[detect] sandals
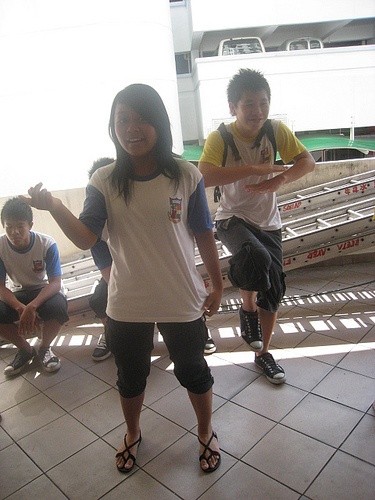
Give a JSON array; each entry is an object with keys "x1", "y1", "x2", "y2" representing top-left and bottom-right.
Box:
[
  {"x1": 114, "y1": 429, "x2": 143, "y2": 474},
  {"x1": 197, "y1": 430, "x2": 221, "y2": 472}
]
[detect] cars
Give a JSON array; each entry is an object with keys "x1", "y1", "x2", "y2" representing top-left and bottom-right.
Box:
[
  {"x1": 218, "y1": 37, "x2": 266, "y2": 56},
  {"x1": 285, "y1": 37, "x2": 323, "y2": 51}
]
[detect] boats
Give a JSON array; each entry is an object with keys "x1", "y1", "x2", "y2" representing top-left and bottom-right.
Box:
[{"x1": 275, "y1": 147, "x2": 375, "y2": 165}]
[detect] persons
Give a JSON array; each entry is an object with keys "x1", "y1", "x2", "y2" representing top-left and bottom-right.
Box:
[
  {"x1": 80, "y1": 158, "x2": 218, "y2": 362},
  {"x1": 19, "y1": 84, "x2": 223, "y2": 476},
  {"x1": 0, "y1": 197, "x2": 70, "y2": 377},
  {"x1": 198, "y1": 68, "x2": 318, "y2": 384}
]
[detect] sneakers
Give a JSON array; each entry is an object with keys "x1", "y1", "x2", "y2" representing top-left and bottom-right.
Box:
[
  {"x1": 254, "y1": 351, "x2": 286, "y2": 384},
  {"x1": 92, "y1": 333, "x2": 111, "y2": 361},
  {"x1": 3, "y1": 346, "x2": 35, "y2": 376},
  {"x1": 37, "y1": 347, "x2": 61, "y2": 372},
  {"x1": 205, "y1": 329, "x2": 217, "y2": 354},
  {"x1": 239, "y1": 305, "x2": 264, "y2": 353}
]
